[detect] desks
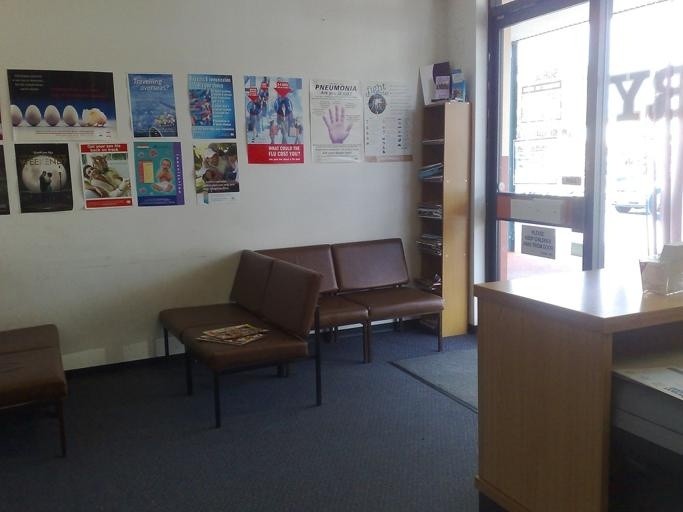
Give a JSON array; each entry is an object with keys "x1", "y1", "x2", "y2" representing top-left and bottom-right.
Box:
[{"x1": 467, "y1": 263, "x2": 682, "y2": 512}]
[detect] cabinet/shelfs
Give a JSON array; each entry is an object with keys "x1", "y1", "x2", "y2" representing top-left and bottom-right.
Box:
[{"x1": 413, "y1": 100, "x2": 470, "y2": 338}]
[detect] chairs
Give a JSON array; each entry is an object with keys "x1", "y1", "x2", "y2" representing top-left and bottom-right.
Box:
[
  {"x1": 254, "y1": 236, "x2": 369, "y2": 364},
  {"x1": 180, "y1": 258, "x2": 325, "y2": 427},
  {"x1": 331, "y1": 238, "x2": 444, "y2": 362},
  {"x1": 158, "y1": 248, "x2": 275, "y2": 398}
]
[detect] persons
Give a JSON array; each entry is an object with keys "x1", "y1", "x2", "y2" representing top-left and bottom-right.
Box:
[
  {"x1": 247, "y1": 92, "x2": 301, "y2": 144},
  {"x1": 84, "y1": 156, "x2": 131, "y2": 197},
  {"x1": 152, "y1": 160, "x2": 174, "y2": 191},
  {"x1": 40, "y1": 171, "x2": 52, "y2": 192},
  {"x1": 322, "y1": 105, "x2": 353, "y2": 144}
]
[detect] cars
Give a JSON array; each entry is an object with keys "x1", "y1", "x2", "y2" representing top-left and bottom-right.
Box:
[{"x1": 609, "y1": 173, "x2": 661, "y2": 218}]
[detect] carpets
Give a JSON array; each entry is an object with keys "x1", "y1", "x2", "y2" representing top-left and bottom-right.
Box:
[{"x1": 387, "y1": 346, "x2": 480, "y2": 415}]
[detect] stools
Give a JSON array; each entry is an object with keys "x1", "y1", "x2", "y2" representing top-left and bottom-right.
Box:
[{"x1": 0, "y1": 323, "x2": 69, "y2": 454}]
